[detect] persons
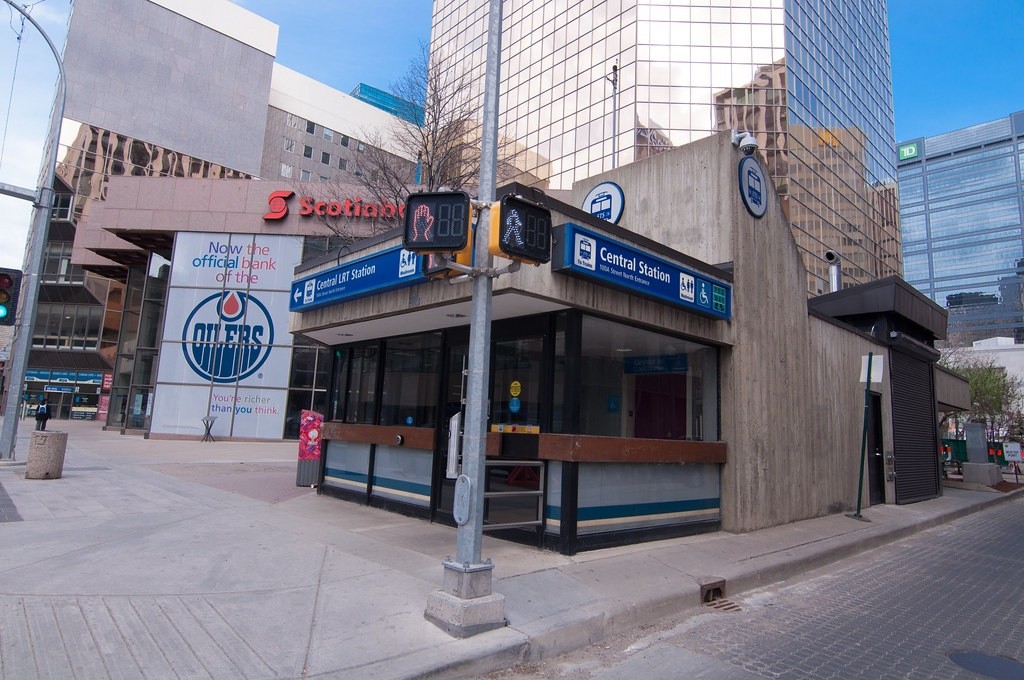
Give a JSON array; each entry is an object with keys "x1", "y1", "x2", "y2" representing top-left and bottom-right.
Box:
[{"x1": 35, "y1": 398, "x2": 51, "y2": 431}]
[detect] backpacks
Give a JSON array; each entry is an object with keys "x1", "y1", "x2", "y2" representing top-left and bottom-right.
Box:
[{"x1": 37, "y1": 404, "x2": 48, "y2": 414}]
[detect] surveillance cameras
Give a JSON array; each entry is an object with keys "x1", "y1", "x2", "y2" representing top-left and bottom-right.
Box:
[{"x1": 739, "y1": 136, "x2": 759, "y2": 156}]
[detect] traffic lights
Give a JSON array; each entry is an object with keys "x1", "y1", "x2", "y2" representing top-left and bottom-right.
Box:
[
  {"x1": 418, "y1": 247, "x2": 472, "y2": 277},
  {"x1": 0, "y1": 267, "x2": 23, "y2": 326},
  {"x1": 488, "y1": 194, "x2": 553, "y2": 266},
  {"x1": 402, "y1": 191, "x2": 473, "y2": 253}
]
